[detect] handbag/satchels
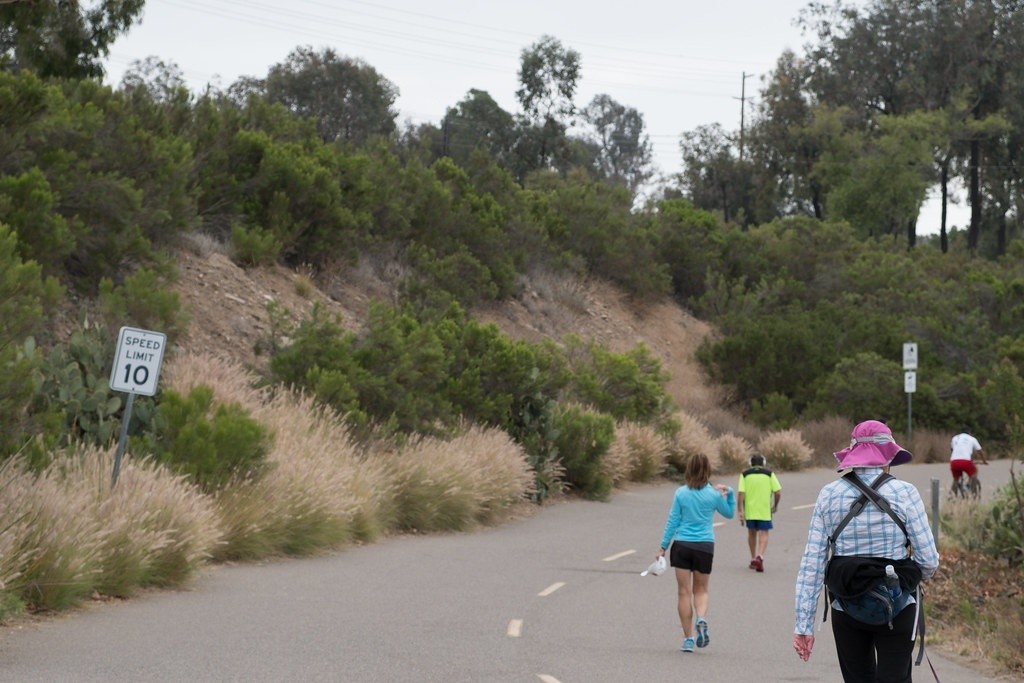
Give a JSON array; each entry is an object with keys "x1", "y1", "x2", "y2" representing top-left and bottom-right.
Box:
[{"x1": 833, "y1": 581, "x2": 910, "y2": 626}]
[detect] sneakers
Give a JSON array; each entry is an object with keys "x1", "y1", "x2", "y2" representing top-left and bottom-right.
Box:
[
  {"x1": 680, "y1": 638, "x2": 694, "y2": 652},
  {"x1": 755, "y1": 556, "x2": 764, "y2": 572},
  {"x1": 750, "y1": 559, "x2": 755, "y2": 569},
  {"x1": 696, "y1": 616, "x2": 710, "y2": 648}
]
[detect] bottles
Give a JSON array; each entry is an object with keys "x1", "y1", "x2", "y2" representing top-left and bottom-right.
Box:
[{"x1": 885, "y1": 564, "x2": 902, "y2": 602}]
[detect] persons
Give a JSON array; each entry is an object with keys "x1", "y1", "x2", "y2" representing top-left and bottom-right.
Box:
[
  {"x1": 949, "y1": 426, "x2": 989, "y2": 496},
  {"x1": 656, "y1": 452, "x2": 736, "y2": 652},
  {"x1": 738, "y1": 453, "x2": 781, "y2": 572},
  {"x1": 793, "y1": 420, "x2": 940, "y2": 683}
]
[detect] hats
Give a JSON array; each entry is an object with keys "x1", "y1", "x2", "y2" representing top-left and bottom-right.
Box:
[
  {"x1": 640, "y1": 556, "x2": 667, "y2": 576},
  {"x1": 833, "y1": 420, "x2": 913, "y2": 474}
]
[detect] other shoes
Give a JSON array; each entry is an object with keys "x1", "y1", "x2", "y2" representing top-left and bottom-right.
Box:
[{"x1": 963, "y1": 483, "x2": 970, "y2": 492}]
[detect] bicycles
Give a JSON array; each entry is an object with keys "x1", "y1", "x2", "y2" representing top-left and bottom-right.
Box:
[{"x1": 948, "y1": 462, "x2": 989, "y2": 501}]
